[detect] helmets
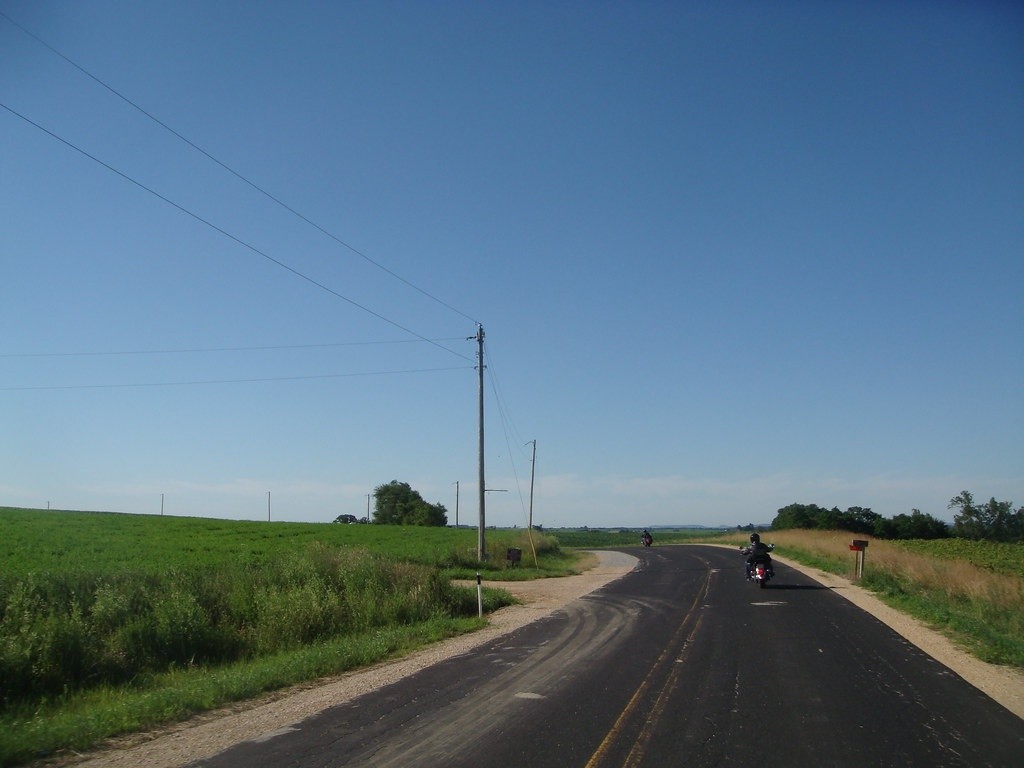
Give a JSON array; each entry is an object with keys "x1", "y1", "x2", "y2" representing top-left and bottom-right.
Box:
[
  {"x1": 643, "y1": 530, "x2": 646, "y2": 532},
  {"x1": 750, "y1": 533, "x2": 760, "y2": 543}
]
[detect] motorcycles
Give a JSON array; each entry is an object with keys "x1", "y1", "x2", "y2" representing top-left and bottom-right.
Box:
[
  {"x1": 641, "y1": 535, "x2": 652, "y2": 547},
  {"x1": 738, "y1": 544, "x2": 776, "y2": 588}
]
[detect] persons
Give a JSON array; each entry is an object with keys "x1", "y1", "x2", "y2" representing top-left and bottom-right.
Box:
[
  {"x1": 641, "y1": 529, "x2": 652, "y2": 543},
  {"x1": 742, "y1": 533, "x2": 773, "y2": 576}
]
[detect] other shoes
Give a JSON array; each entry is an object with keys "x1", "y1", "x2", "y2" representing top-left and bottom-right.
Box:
[{"x1": 744, "y1": 573, "x2": 749, "y2": 576}]
